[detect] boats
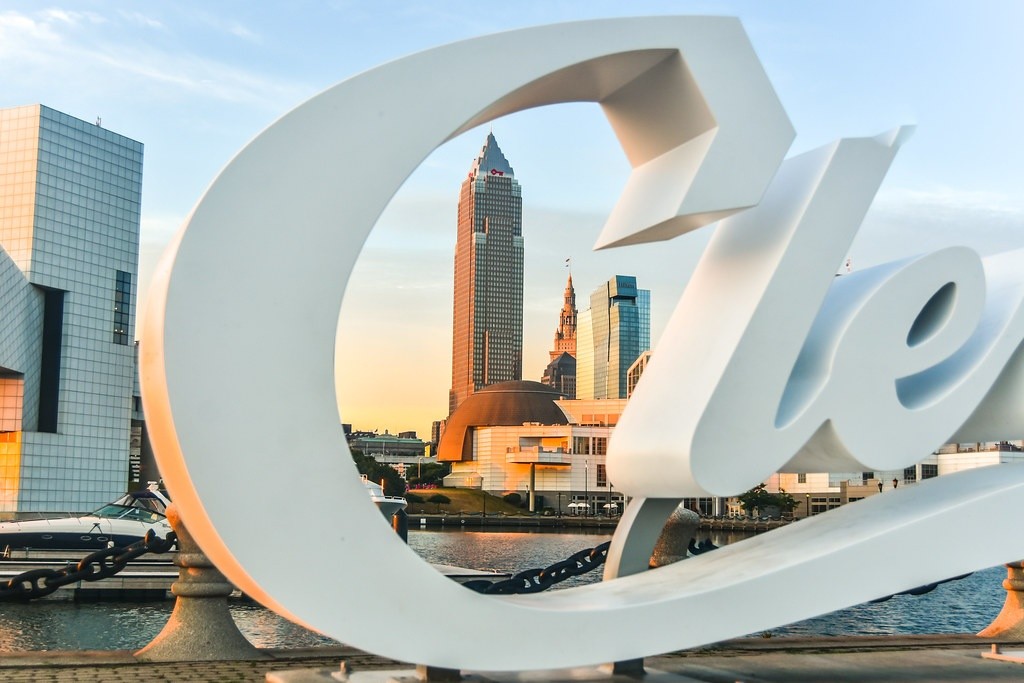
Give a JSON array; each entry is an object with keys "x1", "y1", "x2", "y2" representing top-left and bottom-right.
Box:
[
  {"x1": 427, "y1": 563, "x2": 512, "y2": 584},
  {"x1": 0, "y1": 475, "x2": 409, "y2": 593}
]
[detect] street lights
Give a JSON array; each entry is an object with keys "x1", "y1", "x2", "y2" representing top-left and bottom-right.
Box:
[
  {"x1": 805, "y1": 493, "x2": 811, "y2": 517},
  {"x1": 558, "y1": 492, "x2": 562, "y2": 517},
  {"x1": 609, "y1": 482, "x2": 614, "y2": 518},
  {"x1": 482, "y1": 489, "x2": 486, "y2": 516}
]
